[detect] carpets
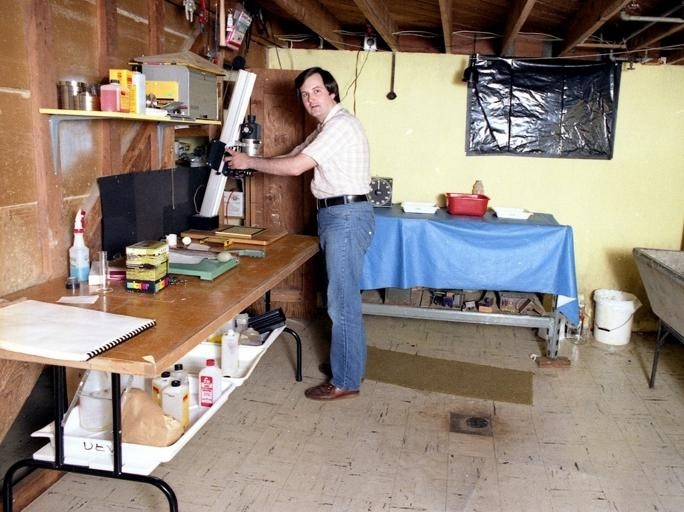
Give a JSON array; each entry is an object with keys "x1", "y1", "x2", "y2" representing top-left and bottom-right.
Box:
[{"x1": 366, "y1": 344, "x2": 534, "y2": 406}]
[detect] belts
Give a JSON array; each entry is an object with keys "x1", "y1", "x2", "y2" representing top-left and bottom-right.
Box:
[{"x1": 316, "y1": 194, "x2": 366, "y2": 210}]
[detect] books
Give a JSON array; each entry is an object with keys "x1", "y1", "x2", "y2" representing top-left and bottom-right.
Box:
[{"x1": 0, "y1": 300, "x2": 157, "y2": 362}]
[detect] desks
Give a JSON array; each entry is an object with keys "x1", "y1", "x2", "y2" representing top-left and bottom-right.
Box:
[
  {"x1": 0, "y1": 232, "x2": 320, "y2": 512},
  {"x1": 360, "y1": 202, "x2": 580, "y2": 367}
]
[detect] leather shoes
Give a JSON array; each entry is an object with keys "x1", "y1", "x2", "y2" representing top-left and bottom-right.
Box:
[
  {"x1": 305, "y1": 381, "x2": 360, "y2": 401},
  {"x1": 319, "y1": 362, "x2": 364, "y2": 382}
]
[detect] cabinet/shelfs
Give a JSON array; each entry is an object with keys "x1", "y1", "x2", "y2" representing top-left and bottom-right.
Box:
[{"x1": 243, "y1": 67, "x2": 324, "y2": 321}]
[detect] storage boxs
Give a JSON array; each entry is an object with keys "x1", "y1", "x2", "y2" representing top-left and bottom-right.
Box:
[{"x1": 142, "y1": 63, "x2": 218, "y2": 120}]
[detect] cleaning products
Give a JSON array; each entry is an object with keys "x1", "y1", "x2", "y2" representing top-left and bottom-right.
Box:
[
  {"x1": 154, "y1": 330, "x2": 240, "y2": 430},
  {"x1": 68, "y1": 209, "x2": 90, "y2": 282},
  {"x1": 132, "y1": 64, "x2": 146, "y2": 114}
]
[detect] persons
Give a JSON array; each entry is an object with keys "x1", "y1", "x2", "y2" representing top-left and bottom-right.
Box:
[{"x1": 225, "y1": 67, "x2": 376, "y2": 400}]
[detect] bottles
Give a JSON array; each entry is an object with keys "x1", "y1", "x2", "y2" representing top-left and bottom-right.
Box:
[
  {"x1": 221, "y1": 329, "x2": 240, "y2": 377},
  {"x1": 130, "y1": 64, "x2": 146, "y2": 115},
  {"x1": 198, "y1": 358, "x2": 222, "y2": 409},
  {"x1": 96, "y1": 250, "x2": 113, "y2": 293},
  {"x1": 472, "y1": 177, "x2": 485, "y2": 194},
  {"x1": 65, "y1": 276, "x2": 80, "y2": 290},
  {"x1": 152, "y1": 363, "x2": 190, "y2": 432},
  {"x1": 567, "y1": 303, "x2": 592, "y2": 345}
]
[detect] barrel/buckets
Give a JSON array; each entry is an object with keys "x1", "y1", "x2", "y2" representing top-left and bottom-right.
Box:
[{"x1": 590, "y1": 289, "x2": 642, "y2": 346}]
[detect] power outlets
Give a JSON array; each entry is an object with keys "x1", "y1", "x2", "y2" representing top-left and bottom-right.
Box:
[{"x1": 363, "y1": 36, "x2": 378, "y2": 50}]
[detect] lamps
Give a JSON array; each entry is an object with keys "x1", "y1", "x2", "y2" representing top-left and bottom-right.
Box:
[{"x1": 462, "y1": 33, "x2": 480, "y2": 83}]
